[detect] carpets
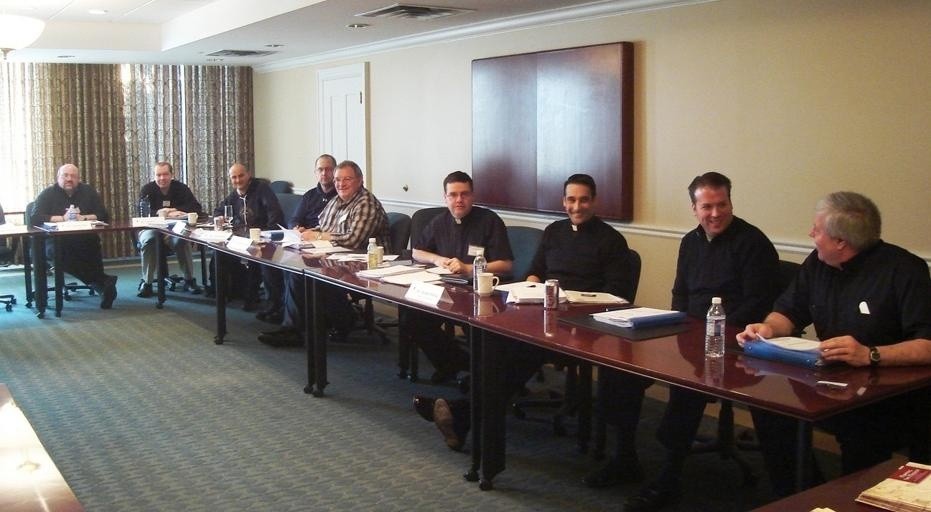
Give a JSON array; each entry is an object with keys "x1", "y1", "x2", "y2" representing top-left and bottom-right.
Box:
[{"x1": 1, "y1": 259, "x2": 837, "y2": 510}]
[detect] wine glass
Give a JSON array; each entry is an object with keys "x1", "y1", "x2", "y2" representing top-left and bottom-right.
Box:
[{"x1": 225, "y1": 205, "x2": 234, "y2": 228}]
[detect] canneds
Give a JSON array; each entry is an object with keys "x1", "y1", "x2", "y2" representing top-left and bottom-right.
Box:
[
  {"x1": 544, "y1": 279, "x2": 559, "y2": 309},
  {"x1": 214, "y1": 216, "x2": 224, "y2": 232}
]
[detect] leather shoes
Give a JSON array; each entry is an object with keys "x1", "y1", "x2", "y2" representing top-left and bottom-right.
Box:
[
  {"x1": 256, "y1": 329, "x2": 304, "y2": 348},
  {"x1": 254, "y1": 305, "x2": 278, "y2": 322},
  {"x1": 430, "y1": 354, "x2": 453, "y2": 385},
  {"x1": 433, "y1": 397, "x2": 468, "y2": 451},
  {"x1": 239, "y1": 297, "x2": 261, "y2": 312},
  {"x1": 183, "y1": 278, "x2": 200, "y2": 294},
  {"x1": 103, "y1": 275, "x2": 116, "y2": 300},
  {"x1": 264, "y1": 305, "x2": 284, "y2": 324},
  {"x1": 136, "y1": 281, "x2": 152, "y2": 297},
  {"x1": 584, "y1": 461, "x2": 644, "y2": 489},
  {"x1": 412, "y1": 395, "x2": 460, "y2": 423},
  {"x1": 100, "y1": 282, "x2": 114, "y2": 309},
  {"x1": 624, "y1": 474, "x2": 682, "y2": 512}
]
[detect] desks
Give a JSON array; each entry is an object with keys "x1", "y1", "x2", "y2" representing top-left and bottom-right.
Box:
[
  {"x1": 158, "y1": 224, "x2": 367, "y2": 393},
  {"x1": 303, "y1": 260, "x2": 559, "y2": 482},
  {"x1": 754, "y1": 452, "x2": 931, "y2": 512},
  {"x1": 1, "y1": 225, "x2": 50, "y2": 319},
  {"x1": 35, "y1": 218, "x2": 209, "y2": 316},
  {"x1": 468, "y1": 303, "x2": 930, "y2": 489}
]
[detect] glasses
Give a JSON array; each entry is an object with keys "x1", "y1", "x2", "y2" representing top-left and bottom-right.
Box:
[
  {"x1": 445, "y1": 191, "x2": 471, "y2": 198},
  {"x1": 315, "y1": 167, "x2": 333, "y2": 173},
  {"x1": 58, "y1": 173, "x2": 79, "y2": 180},
  {"x1": 332, "y1": 176, "x2": 358, "y2": 185}
]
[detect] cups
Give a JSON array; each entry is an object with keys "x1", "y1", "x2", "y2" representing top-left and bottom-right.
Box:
[
  {"x1": 249, "y1": 228, "x2": 261, "y2": 243},
  {"x1": 159, "y1": 210, "x2": 167, "y2": 220},
  {"x1": 377, "y1": 246, "x2": 385, "y2": 264},
  {"x1": 187, "y1": 212, "x2": 199, "y2": 226},
  {"x1": 213, "y1": 216, "x2": 223, "y2": 231},
  {"x1": 476, "y1": 273, "x2": 499, "y2": 297}
]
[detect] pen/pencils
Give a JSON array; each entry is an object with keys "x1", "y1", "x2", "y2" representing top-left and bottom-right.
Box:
[
  {"x1": 525, "y1": 284, "x2": 538, "y2": 287},
  {"x1": 580, "y1": 293, "x2": 596, "y2": 297}
]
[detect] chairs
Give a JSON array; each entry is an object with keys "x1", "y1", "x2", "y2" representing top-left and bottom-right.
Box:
[
  {"x1": 1, "y1": 206, "x2": 17, "y2": 311},
  {"x1": 346, "y1": 211, "x2": 411, "y2": 339},
  {"x1": 686, "y1": 260, "x2": 802, "y2": 487},
  {"x1": 436, "y1": 226, "x2": 549, "y2": 420},
  {"x1": 272, "y1": 193, "x2": 303, "y2": 231},
  {"x1": 512, "y1": 249, "x2": 642, "y2": 435},
  {"x1": 132, "y1": 227, "x2": 188, "y2": 291},
  {"x1": 25, "y1": 202, "x2": 96, "y2": 300},
  {"x1": 410, "y1": 209, "x2": 447, "y2": 364}
]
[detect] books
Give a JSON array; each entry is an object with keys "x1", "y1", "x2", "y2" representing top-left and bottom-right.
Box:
[
  {"x1": 496, "y1": 279, "x2": 688, "y2": 328},
  {"x1": 855, "y1": 460, "x2": 931, "y2": 511}
]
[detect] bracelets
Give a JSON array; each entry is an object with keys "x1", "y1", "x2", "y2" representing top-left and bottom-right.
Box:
[
  {"x1": 82, "y1": 214, "x2": 88, "y2": 221},
  {"x1": 62, "y1": 215, "x2": 65, "y2": 222}
]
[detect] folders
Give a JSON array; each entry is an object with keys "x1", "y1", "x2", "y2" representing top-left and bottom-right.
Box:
[
  {"x1": 589, "y1": 302, "x2": 687, "y2": 329},
  {"x1": 744, "y1": 330, "x2": 843, "y2": 371}
]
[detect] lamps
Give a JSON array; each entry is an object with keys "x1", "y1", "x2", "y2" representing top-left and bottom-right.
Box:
[{"x1": 2, "y1": 12, "x2": 46, "y2": 62}]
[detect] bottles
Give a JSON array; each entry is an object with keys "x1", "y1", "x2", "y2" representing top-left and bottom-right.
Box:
[
  {"x1": 704, "y1": 297, "x2": 727, "y2": 358},
  {"x1": 367, "y1": 237, "x2": 378, "y2": 269},
  {"x1": 140, "y1": 198, "x2": 151, "y2": 218},
  {"x1": 472, "y1": 249, "x2": 487, "y2": 292},
  {"x1": 68, "y1": 204, "x2": 78, "y2": 230}
]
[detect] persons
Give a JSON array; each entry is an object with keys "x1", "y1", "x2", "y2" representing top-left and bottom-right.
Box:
[
  {"x1": 276, "y1": 154, "x2": 339, "y2": 326},
  {"x1": 398, "y1": 170, "x2": 515, "y2": 392},
  {"x1": 136, "y1": 163, "x2": 201, "y2": 296},
  {"x1": 737, "y1": 193, "x2": 931, "y2": 497},
  {"x1": 582, "y1": 171, "x2": 778, "y2": 507},
  {"x1": 30, "y1": 162, "x2": 117, "y2": 309},
  {"x1": 258, "y1": 159, "x2": 392, "y2": 345},
  {"x1": 413, "y1": 174, "x2": 631, "y2": 451},
  {"x1": 213, "y1": 164, "x2": 288, "y2": 323}
]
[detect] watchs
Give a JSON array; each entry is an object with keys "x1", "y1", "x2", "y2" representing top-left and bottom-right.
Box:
[
  {"x1": 869, "y1": 340, "x2": 881, "y2": 369},
  {"x1": 318, "y1": 231, "x2": 323, "y2": 241}
]
